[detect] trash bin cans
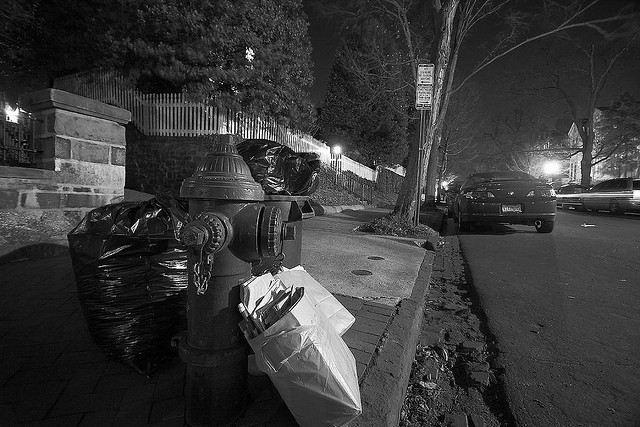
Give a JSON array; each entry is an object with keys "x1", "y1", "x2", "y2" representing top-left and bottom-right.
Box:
[{"x1": 265, "y1": 194, "x2": 325, "y2": 268}]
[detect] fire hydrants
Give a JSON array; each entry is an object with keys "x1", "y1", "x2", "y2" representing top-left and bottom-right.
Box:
[{"x1": 178, "y1": 136, "x2": 297, "y2": 422}]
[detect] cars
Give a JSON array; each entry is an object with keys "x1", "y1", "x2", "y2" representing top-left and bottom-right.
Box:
[
  {"x1": 446, "y1": 180, "x2": 463, "y2": 218},
  {"x1": 452, "y1": 171, "x2": 557, "y2": 233},
  {"x1": 557, "y1": 185, "x2": 588, "y2": 211},
  {"x1": 581, "y1": 178, "x2": 640, "y2": 217}
]
